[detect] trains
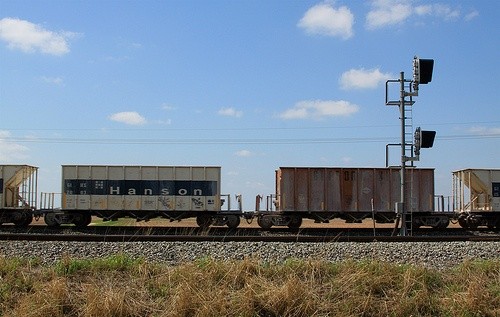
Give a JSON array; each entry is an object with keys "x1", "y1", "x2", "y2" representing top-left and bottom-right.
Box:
[{"x1": 0, "y1": 165, "x2": 500, "y2": 229}]
